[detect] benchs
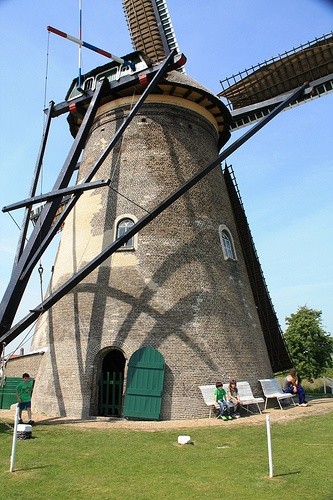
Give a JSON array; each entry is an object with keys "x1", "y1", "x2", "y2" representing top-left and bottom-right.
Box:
[
  {"x1": 258, "y1": 379, "x2": 296, "y2": 410},
  {"x1": 198, "y1": 381, "x2": 264, "y2": 419}
]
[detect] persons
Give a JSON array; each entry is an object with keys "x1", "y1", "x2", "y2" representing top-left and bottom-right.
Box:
[
  {"x1": 227, "y1": 380, "x2": 240, "y2": 418},
  {"x1": 282, "y1": 369, "x2": 311, "y2": 407},
  {"x1": 214, "y1": 382, "x2": 232, "y2": 421},
  {"x1": 16, "y1": 373, "x2": 34, "y2": 424}
]
